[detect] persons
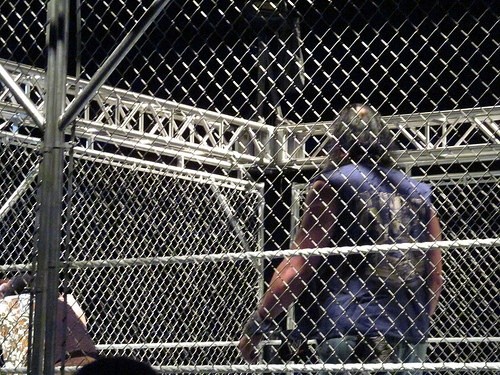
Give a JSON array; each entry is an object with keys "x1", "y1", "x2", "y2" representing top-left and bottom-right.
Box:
[
  {"x1": 238, "y1": 102, "x2": 444, "y2": 366},
  {"x1": 0, "y1": 271, "x2": 155, "y2": 375}
]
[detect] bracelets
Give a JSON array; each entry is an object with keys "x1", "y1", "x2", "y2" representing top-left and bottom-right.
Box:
[{"x1": 246, "y1": 309, "x2": 271, "y2": 337}]
